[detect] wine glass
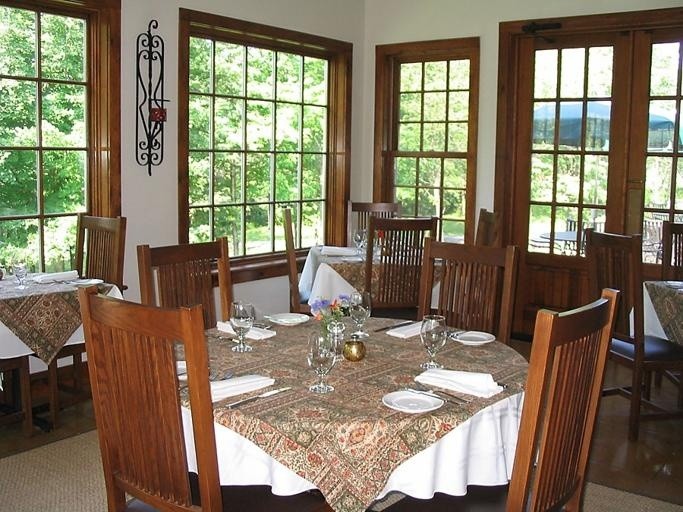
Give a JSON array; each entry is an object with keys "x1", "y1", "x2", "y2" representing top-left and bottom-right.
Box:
[
  {"x1": 352, "y1": 227, "x2": 365, "y2": 258},
  {"x1": 227, "y1": 299, "x2": 257, "y2": 354},
  {"x1": 347, "y1": 291, "x2": 372, "y2": 339},
  {"x1": 10, "y1": 256, "x2": 30, "y2": 291},
  {"x1": 419, "y1": 314, "x2": 451, "y2": 373},
  {"x1": 306, "y1": 329, "x2": 338, "y2": 396}
]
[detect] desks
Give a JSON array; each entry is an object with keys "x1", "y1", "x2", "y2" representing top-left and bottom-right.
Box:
[{"x1": 540, "y1": 231, "x2": 589, "y2": 256}]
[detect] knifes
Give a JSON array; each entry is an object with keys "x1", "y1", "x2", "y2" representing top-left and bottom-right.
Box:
[
  {"x1": 374, "y1": 322, "x2": 413, "y2": 333},
  {"x1": 222, "y1": 386, "x2": 291, "y2": 409}
]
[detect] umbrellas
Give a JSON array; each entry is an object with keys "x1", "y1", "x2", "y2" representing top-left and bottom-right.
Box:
[{"x1": 533, "y1": 102, "x2": 674, "y2": 229}]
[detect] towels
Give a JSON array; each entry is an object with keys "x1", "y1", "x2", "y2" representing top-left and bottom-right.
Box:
[
  {"x1": 209, "y1": 374, "x2": 275, "y2": 404},
  {"x1": 414, "y1": 368, "x2": 503, "y2": 400},
  {"x1": 30, "y1": 270, "x2": 81, "y2": 285},
  {"x1": 216, "y1": 320, "x2": 277, "y2": 342}
]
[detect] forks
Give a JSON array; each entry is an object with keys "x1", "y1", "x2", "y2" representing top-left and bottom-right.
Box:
[{"x1": 176, "y1": 371, "x2": 218, "y2": 392}]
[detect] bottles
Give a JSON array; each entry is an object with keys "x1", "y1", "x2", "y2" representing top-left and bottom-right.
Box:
[
  {"x1": 0, "y1": 266, "x2": 7, "y2": 279},
  {"x1": 342, "y1": 333, "x2": 366, "y2": 361}
]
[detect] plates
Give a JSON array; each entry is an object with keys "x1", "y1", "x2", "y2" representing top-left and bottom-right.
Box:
[
  {"x1": 380, "y1": 388, "x2": 443, "y2": 416},
  {"x1": 268, "y1": 311, "x2": 312, "y2": 327},
  {"x1": 64, "y1": 277, "x2": 103, "y2": 288},
  {"x1": 449, "y1": 330, "x2": 496, "y2": 348},
  {"x1": 663, "y1": 278, "x2": 682, "y2": 290},
  {"x1": 341, "y1": 256, "x2": 366, "y2": 263}
]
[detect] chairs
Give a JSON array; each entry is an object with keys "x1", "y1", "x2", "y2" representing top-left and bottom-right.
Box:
[
  {"x1": 585, "y1": 228, "x2": 683, "y2": 443},
  {"x1": 77, "y1": 283, "x2": 224, "y2": 512},
  {"x1": 0, "y1": 357, "x2": 34, "y2": 439},
  {"x1": 136, "y1": 237, "x2": 232, "y2": 330},
  {"x1": 565, "y1": 201, "x2": 682, "y2": 264},
  {"x1": 654, "y1": 221, "x2": 683, "y2": 408},
  {"x1": 567, "y1": 201, "x2": 683, "y2": 265},
  {"x1": 282, "y1": 200, "x2": 518, "y2": 345},
  {"x1": 505, "y1": 287, "x2": 621, "y2": 512},
  {"x1": 31, "y1": 214, "x2": 127, "y2": 431}
]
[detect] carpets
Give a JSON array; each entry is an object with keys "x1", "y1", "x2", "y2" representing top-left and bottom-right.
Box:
[{"x1": 0, "y1": 430, "x2": 683, "y2": 512}]
